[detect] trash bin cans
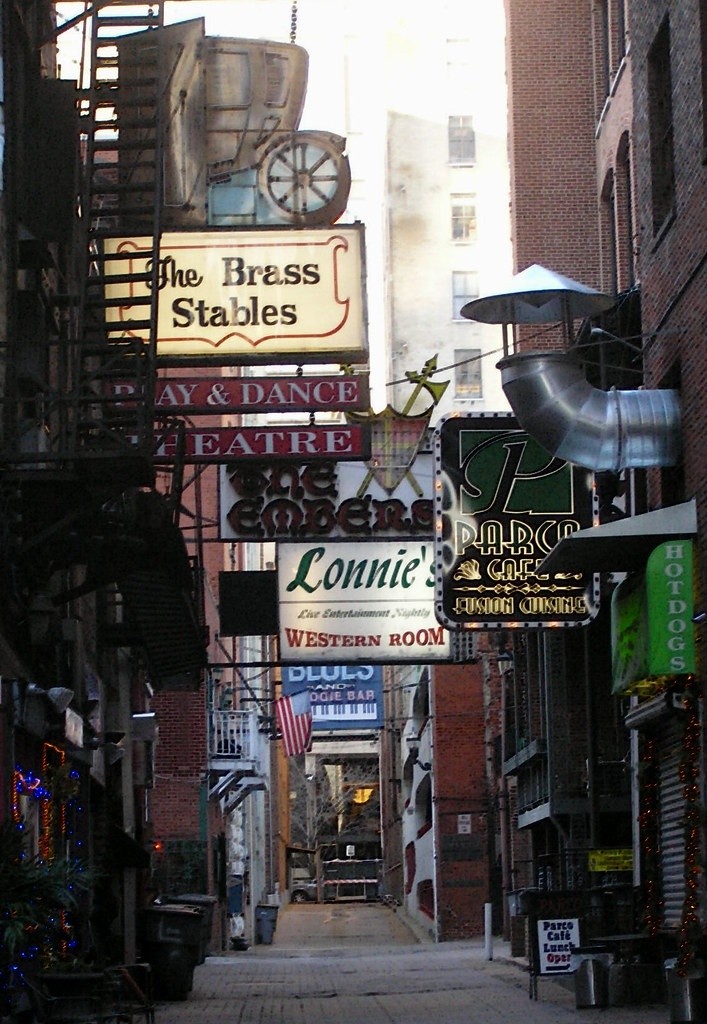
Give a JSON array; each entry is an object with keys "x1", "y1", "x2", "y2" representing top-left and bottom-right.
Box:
[
  {"x1": 137, "y1": 892, "x2": 218, "y2": 1003},
  {"x1": 254, "y1": 906, "x2": 279, "y2": 946}
]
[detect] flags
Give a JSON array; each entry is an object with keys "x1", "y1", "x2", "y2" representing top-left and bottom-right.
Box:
[{"x1": 276, "y1": 686, "x2": 313, "y2": 758}]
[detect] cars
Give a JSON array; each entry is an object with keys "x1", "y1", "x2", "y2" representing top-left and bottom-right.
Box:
[{"x1": 292, "y1": 877, "x2": 316, "y2": 903}]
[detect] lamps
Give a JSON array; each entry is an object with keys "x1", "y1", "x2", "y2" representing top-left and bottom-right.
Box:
[
  {"x1": 85, "y1": 736, "x2": 125, "y2": 766},
  {"x1": 18, "y1": 680, "x2": 74, "y2": 714}
]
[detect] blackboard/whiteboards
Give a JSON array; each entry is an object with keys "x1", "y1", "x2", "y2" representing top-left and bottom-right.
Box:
[{"x1": 529, "y1": 913, "x2": 585, "y2": 976}]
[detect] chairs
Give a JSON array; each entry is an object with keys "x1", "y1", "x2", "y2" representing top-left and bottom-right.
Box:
[{"x1": 20, "y1": 963, "x2": 156, "y2": 1024}]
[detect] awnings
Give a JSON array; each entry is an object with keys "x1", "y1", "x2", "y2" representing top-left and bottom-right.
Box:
[{"x1": 534, "y1": 496, "x2": 697, "y2": 575}]
